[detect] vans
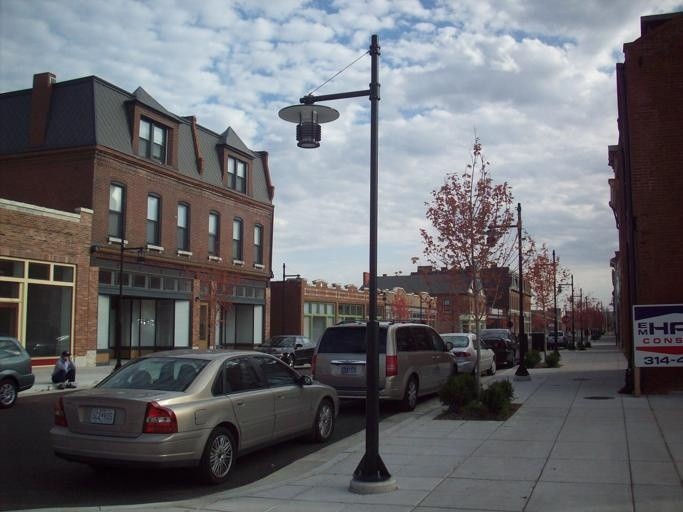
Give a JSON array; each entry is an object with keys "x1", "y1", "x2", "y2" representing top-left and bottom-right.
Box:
[{"x1": 310, "y1": 320, "x2": 457, "y2": 412}]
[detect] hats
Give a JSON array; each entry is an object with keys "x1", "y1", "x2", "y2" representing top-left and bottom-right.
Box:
[{"x1": 62, "y1": 351, "x2": 70, "y2": 356}]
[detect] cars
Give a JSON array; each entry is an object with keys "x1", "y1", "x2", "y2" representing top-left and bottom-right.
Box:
[
  {"x1": 48, "y1": 350, "x2": 339, "y2": 484},
  {"x1": 254, "y1": 334, "x2": 316, "y2": 369},
  {"x1": 1, "y1": 337, "x2": 34, "y2": 407},
  {"x1": 439, "y1": 328, "x2": 521, "y2": 378}
]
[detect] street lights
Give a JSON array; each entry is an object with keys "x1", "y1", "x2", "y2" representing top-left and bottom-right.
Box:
[
  {"x1": 115, "y1": 244, "x2": 146, "y2": 368},
  {"x1": 534, "y1": 250, "x2": 586, "y2": 342},
  {"x1": 484, "y1": 203, "x2": 529, "y2": 376}
]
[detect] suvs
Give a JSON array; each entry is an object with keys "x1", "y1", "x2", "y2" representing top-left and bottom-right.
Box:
[{"x1": 547, "y1": 330, "x2": 568, "y2": 350}]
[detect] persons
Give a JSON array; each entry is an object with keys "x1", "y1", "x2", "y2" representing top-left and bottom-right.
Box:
[{"x1": 51, "y1": 350, "x2": 77, "y2": 389}]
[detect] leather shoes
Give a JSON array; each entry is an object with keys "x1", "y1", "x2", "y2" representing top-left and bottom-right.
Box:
[
  {"x1": 65, "y1": 383, "x2": 76, "y2": 388},
  {"x1": 58, "y1": 384, "x2": 64, "y2": 389}
]
